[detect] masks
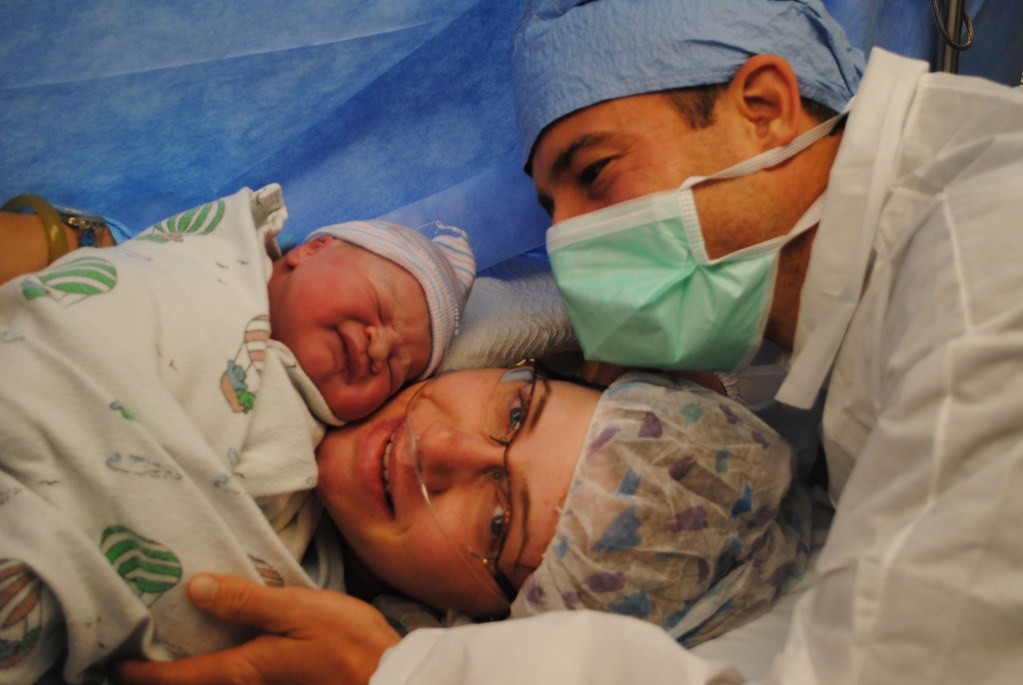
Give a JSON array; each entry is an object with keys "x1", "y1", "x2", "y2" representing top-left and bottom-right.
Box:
[{"x1": 545, "y1": 146, "x2": 779, "y2": 373}]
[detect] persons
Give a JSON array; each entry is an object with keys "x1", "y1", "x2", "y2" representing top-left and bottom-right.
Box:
[
  {"x1": 119, "y1": 0, "x2": 1023, "y2": 685},
  {"x1": 0, "y1": 212, "x2": 798, "y2": 643},
  {"x1": 1, "y1": 180, "x2": 474, "y2": 685}
]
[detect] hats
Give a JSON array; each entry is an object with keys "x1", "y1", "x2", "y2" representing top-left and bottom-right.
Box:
[
  {"x1": 302, "y1": 218, "x2": 475, "y2": 380},
  {"x1": 513, "y1": 0, "x2": 865, "y2": 174}
]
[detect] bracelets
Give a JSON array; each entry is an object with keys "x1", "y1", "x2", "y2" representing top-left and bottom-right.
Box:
[{"x1": 0, "y1": 194, "x2": 69, "y2": 267}]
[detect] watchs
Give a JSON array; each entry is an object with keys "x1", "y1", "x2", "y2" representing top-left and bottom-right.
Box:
[{"x1": 61, "y1": 211, "x2": 109, "y2": 247}]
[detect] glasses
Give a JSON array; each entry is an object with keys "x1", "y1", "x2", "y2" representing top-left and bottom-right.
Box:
[{"x1": 467, "y1": 356, "x2": 544, "y2": 602}]
